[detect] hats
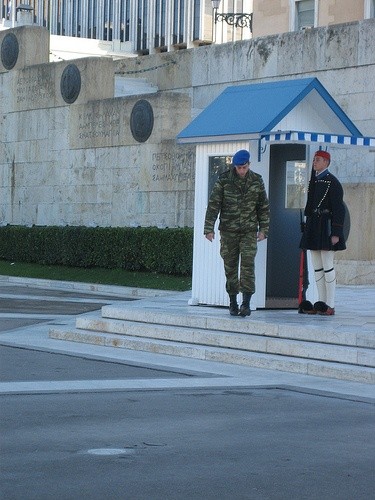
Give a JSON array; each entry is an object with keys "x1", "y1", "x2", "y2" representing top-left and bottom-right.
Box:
[
  {"x1": 315, "y1": 151, "x2": 330, "y2": 159},
  {"x1": 233, "y1": 150, "x2": 250, "y2": 165}
]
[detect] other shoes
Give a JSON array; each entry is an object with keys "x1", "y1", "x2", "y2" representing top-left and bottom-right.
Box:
[{"x1": 299, "y1": 301, "x2": 335, "y2": 315}]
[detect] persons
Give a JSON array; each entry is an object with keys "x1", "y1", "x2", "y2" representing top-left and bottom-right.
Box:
[
  {"x1": 299, "y1": 151, "x2": 347, "y2": 315},
  {"x1": 203, "y1": 149, "x2": 271, "y2": 317}
]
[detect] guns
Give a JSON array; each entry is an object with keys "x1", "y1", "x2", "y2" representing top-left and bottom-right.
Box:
[{"x1": 298, "y1": 208, "x2": 310, "y2": 304}]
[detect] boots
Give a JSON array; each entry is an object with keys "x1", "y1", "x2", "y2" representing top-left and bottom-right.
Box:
[{"x1": 230, "y1": 293, "x2": 252, "y2": 317}]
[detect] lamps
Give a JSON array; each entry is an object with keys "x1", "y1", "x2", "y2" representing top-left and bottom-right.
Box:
[{"x1": 211, "y1": 0, "x2": 253, "y2": 33}]
